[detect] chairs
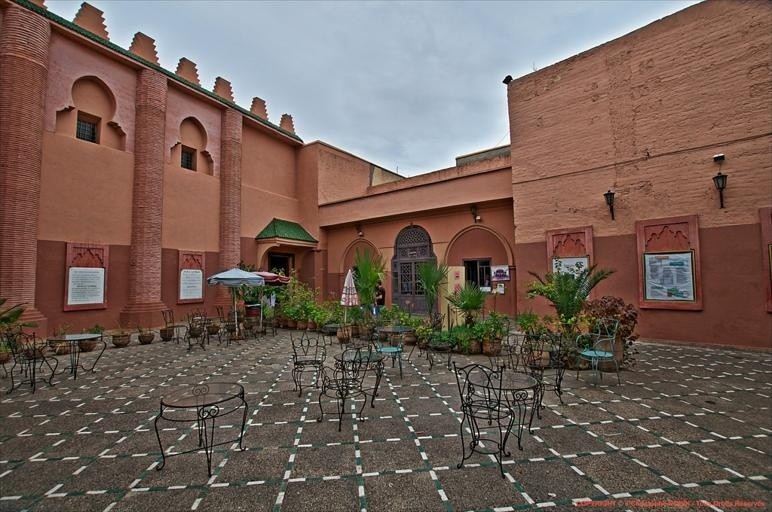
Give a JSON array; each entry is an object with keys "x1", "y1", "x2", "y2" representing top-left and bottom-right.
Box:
[{"x1": 0, "y1": 300, "x2": 622, "y2": 480}]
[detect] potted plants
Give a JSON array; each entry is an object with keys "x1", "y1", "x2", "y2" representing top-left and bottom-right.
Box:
[{"x1": 232, "y1": 260, "x2": 636, "y2": 368}]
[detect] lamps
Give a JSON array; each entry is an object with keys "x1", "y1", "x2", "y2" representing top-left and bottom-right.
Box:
[
  {"x1": 471, "y1": 204, "x2": 482, "y2": 223},
  {"x1": 712, "y1": 171, "x2": 729, "y2": 209},
  {"x1": 603, "y1": 190, "x2": 616, "y2": 219}
]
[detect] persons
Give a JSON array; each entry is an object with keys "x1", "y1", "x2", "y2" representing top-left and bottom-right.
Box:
[{"x1": 375, "y1": 281, "x2": 385, "y2": 310}]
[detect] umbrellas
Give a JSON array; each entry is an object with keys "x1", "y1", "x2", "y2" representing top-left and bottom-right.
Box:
[
  {"x1": 340, "y1": 268, "x2": 359, "y2": 323},
  {"x1": 206, "y1": 268, "x2": 265, "y2": 336},
  {"x1": 250, "y1": 271, "x2": 291, "y2": 321}
]
